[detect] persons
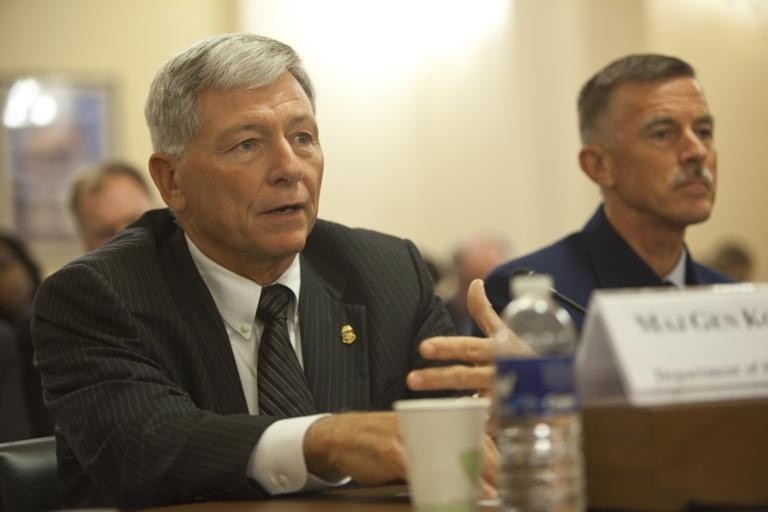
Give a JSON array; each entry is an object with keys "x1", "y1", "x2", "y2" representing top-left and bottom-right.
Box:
[
  {"x1": 18, "y1": 155, "x2": 163, "y2": 437},
  {"x1": 465, "y1": 51, "x2": 752, "y2": 418},
  {"x1": 1, "y1": 235, "x2": 42, "y2": 320},
  {"x1": 32, "y1": 35, "x2": 545, "y2": 499}
]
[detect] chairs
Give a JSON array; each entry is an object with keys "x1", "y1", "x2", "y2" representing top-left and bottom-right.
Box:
[{"x1": 1, "y1": 433, "x2": 63, "y2": 510}]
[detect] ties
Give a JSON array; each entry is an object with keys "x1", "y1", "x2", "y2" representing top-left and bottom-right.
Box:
[{"x1": 249, "y1": 283, "x2": 320, "y2": 416}]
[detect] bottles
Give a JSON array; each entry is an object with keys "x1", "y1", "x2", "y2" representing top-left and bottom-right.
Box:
[{"x1": 490, "y1": 273, "x2": 587, "y2": 512}]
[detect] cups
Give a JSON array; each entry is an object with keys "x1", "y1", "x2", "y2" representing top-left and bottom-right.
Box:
[{"x1": 392, "y1": 398, "x2": 488, "y2": 511}]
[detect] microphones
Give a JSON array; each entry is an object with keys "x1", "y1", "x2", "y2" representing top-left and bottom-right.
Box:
[{"x1": 512, "y1": 267, "x2": 587, "y2": 320}]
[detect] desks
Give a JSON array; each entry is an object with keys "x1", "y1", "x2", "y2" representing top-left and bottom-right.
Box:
[{"x1": 134, "y1": 484, "x2": 412, "y2": 512}]
